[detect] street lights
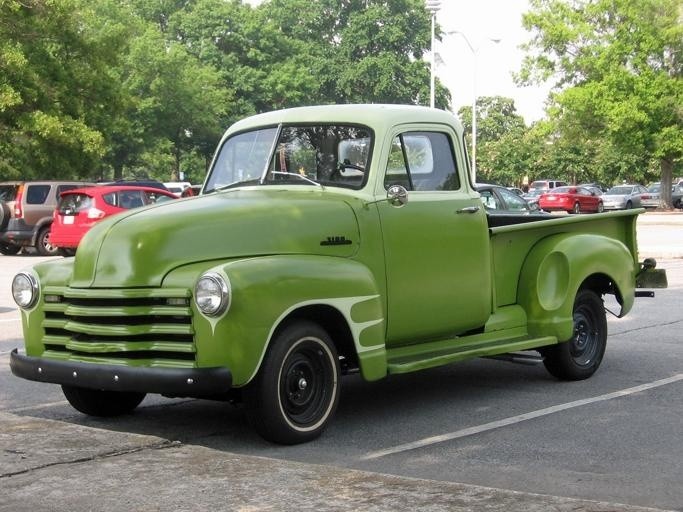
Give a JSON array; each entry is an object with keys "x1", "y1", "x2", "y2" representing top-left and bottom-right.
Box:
[
  {"x1": 446, "y1": 30, "x2": 478, "y2": 189},
  {"x1": 422, "y1": 2, "x2": 441, "y2": 107}
]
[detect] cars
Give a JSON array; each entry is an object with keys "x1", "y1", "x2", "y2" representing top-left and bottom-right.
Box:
[
  {"x1": 475, "y1": 179, "x2": 682, "y2": 216},
  {"x1": 0, "y1": 176, "x2": 207, "y2": 257}
]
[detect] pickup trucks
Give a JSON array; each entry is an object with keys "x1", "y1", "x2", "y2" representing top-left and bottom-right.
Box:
[{"x1": 7, "y1": 104, "x2": 646, "y2": 446}]
